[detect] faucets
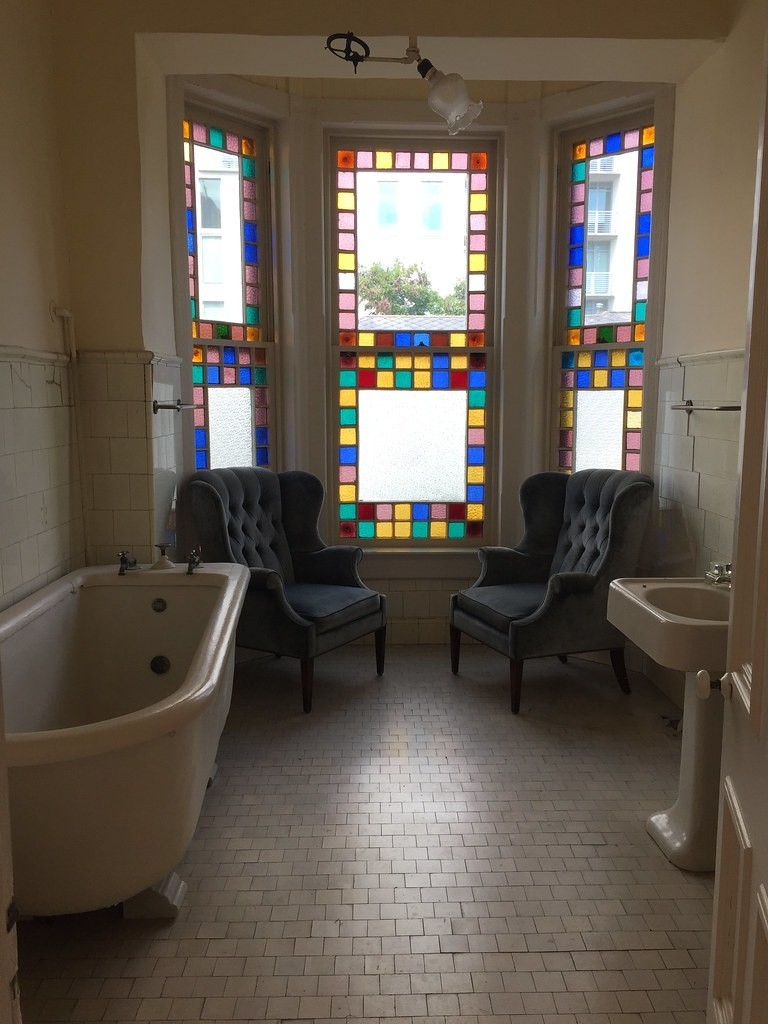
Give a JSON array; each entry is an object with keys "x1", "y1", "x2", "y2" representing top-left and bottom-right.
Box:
[
  {"x1": 185, "y1": 545, "x2": 204, "y2": 575},
  {"x1": 702, "y1": 562, "x2": 733, "y2": 586},
  {"x1": 117, "y1": 550, "x2": 137, "y2": 576}
]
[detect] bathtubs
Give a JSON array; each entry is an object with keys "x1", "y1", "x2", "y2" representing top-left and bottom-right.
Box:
[{"x1": 0, "y1": 562, "x2": 251, "y2": 917}]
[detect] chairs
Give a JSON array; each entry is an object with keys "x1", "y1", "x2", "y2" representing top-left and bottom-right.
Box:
[
  {"x1": 187, "y1": 466, "x2": 389, "y2": 716},
  {"x1": 448, "y1": 469, "x2": 657, "y2": 716}
]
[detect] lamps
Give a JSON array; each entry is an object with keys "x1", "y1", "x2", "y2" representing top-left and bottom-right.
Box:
[{"x1": 415, "y1": 57, "x2": 483, "y2": 135}]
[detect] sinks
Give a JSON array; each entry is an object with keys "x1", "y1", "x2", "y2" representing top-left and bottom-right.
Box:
[{"x1": 606, "y1": 577, "x2": 731, "y2": 671}]
